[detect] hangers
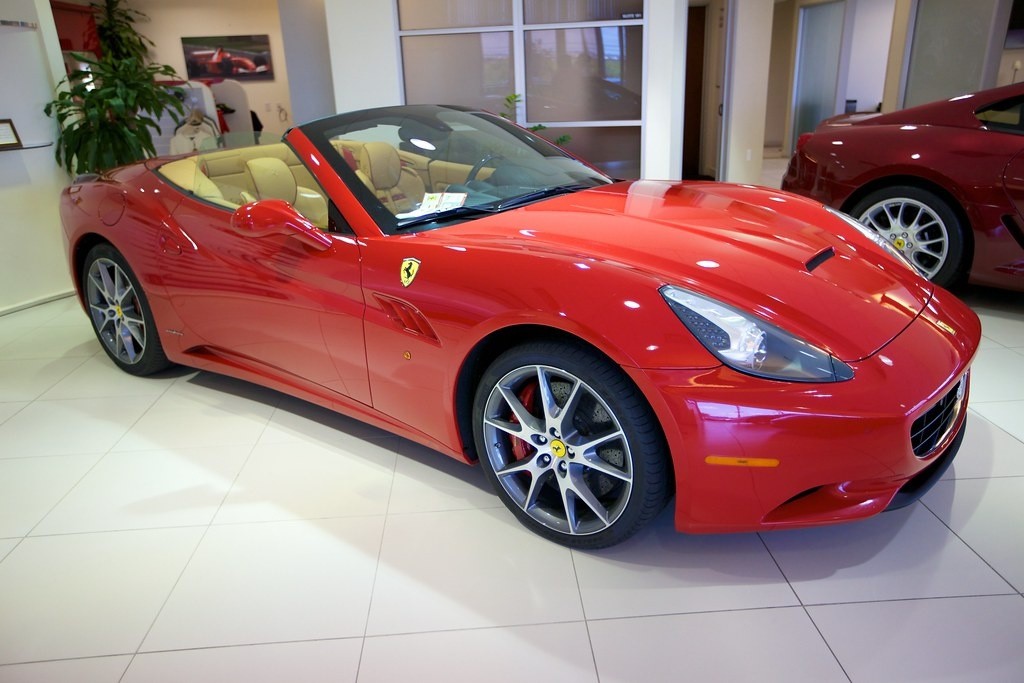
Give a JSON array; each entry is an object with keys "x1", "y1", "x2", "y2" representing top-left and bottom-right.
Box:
[{"x1": 175, "y1": 107, "x2": 215, "y2": 135}]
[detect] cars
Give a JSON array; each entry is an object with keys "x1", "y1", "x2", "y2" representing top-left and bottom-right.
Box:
[
  {"x1": 779, "y1": 80, "x2": 1024, "y2": 311},
  {"x1": 58, "y1": 98, "x2": 985, "y2": 551},
  {"x1": 187, "y1": 45, "x2": 274, "y2": 80}
]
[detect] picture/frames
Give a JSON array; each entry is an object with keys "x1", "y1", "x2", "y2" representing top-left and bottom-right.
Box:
[{"x1": 0, "y1": 118, "x2": 23, "y2": 149}]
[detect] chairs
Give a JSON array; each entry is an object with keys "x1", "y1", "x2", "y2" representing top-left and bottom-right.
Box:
[
  {"x1": 236, "y1": 155, "x2": 329, "y2": 229},
  {"x1": 352, "y1": 139, "x2": 428, "y2": 216}
]
